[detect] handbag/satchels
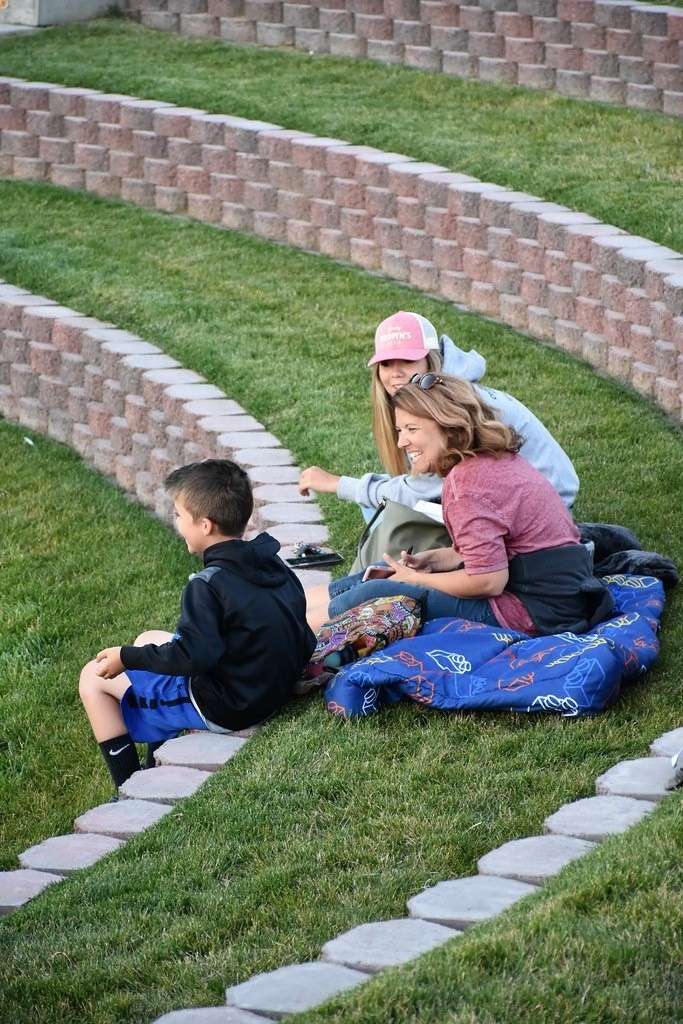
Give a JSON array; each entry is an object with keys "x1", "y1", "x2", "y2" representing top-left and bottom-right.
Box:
[
  {"x1": 347, "y1": 496, "x2": 452, "y2": 576},
  {"x1": 294, "y1": 590, "x2": 428, "y2": 696}
]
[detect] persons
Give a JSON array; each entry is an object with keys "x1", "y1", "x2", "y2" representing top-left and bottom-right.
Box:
[
  {"x1": 305, "y1": 372, "x2": 581, "y2": 637},
  {"x1": 79, "y1": 459, "x2": 317, "y2": 796},
  {"x1": 298, "y1": 310, "x2": 581, "y2": 518}
]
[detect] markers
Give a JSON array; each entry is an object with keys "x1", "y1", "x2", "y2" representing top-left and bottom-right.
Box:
[{"x1": 403, "y1": 545, "x2": 414, "y2": 566}]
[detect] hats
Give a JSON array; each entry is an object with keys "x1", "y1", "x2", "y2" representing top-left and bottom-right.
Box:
[{"x1": 367, "y1": 310, "x2": 439, "y2": 367}]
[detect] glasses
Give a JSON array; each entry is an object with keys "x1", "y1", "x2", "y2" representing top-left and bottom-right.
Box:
[{"x1": 409, "y1": 373, "x2": 447, "y2": 390}]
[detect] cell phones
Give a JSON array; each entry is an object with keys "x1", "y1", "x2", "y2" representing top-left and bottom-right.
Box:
[
  {"x1": 362, "y1": 566, "x2": 396, "y2": 583},
  {"x1": 288, "y1": 552, "x2": 346, "y2": 566}
]
